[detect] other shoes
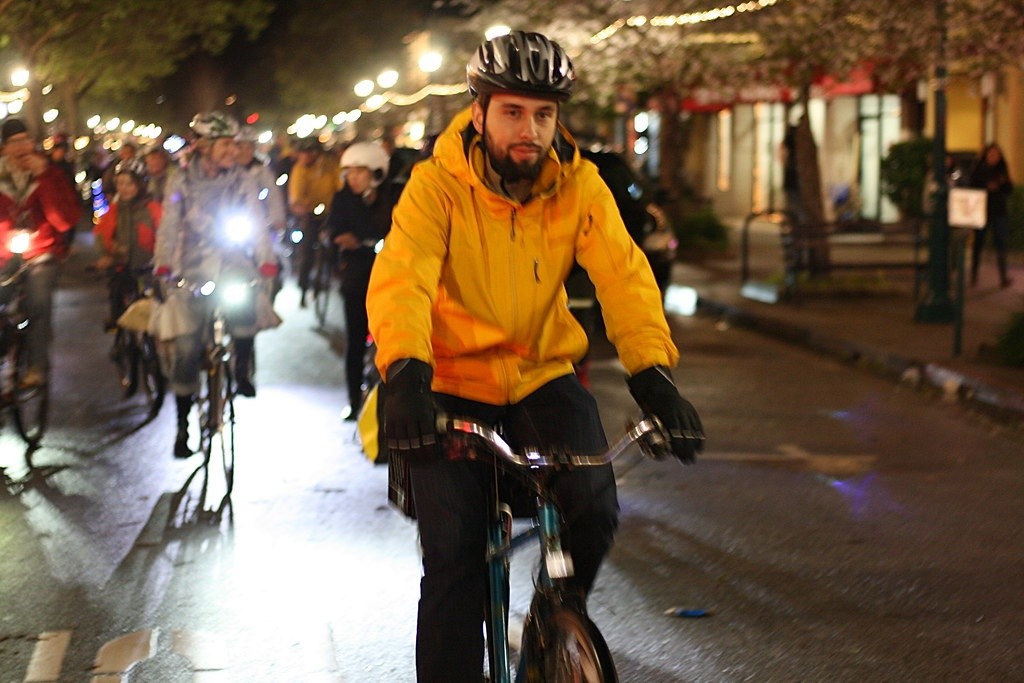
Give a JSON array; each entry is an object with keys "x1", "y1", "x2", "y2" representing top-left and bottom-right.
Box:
[
  {"x1": 15, "y1": 370, "x2": 42, "y2": 400},
  {"x1": 174, "y1": 423, "x2": 188, "y2": 458},
  {"x1": 234, "y1": 368, "x2": 257, "y2": 396}
]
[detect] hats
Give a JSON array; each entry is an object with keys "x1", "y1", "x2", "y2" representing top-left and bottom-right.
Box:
[{"x1": 0, "y1": 116, "x2": 26, "y2": 143}]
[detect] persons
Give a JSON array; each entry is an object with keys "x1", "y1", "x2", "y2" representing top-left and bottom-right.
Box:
[
  {"x1": 969, "y1": 142, "x2": 1015, "y2": 288},
  {"x1": 355, "y1": 30, "x2": 705, "y2": 683},
  {"x1": 0, "y1": 109, "x2": 434, "y2": 459}
]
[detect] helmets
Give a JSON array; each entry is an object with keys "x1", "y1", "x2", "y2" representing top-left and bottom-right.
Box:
[
  {"x1": 114, "y1": 158, "x2": 149, "y2": 182},
  {"x1": 466, "y1": 31, "x2": 574, "y2": 105},
  {"x1": 230, "y1": 125, "x2": 260, "y2": 143},
  {"x1": 338, "y1": 140, "x2": 391, "y2": 187},
  {"x1": 189, "y1": 110, "x2": 239, "y2": 138}
]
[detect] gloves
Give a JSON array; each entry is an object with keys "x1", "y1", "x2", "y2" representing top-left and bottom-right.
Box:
[
  {"x1": 625, "y1": 363, "x2": 707, "y2": 466},
  {"x1": 376, "y1": 358, "x2": 444, "y2": 464}
]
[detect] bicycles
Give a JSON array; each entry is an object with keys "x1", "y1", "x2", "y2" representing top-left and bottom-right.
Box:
[
  {"x1": 358, "y1": 237, "x2": 382, "y2": 398},
  {"x1": 174, "y1": 277, "x2": 265, "y2": 492},
  {"x1": 109, "y1": 263, "x2": 168, "y2": 408},
  {"x1": 431, "y1": 411, "x2": 666, "y2": 683},
  {"x1": 300, "y1": 211, "x2": 332, "y2": 316},
  {"x1": 0, "y1": 253, "x2": 52, "y2": 445}
]
